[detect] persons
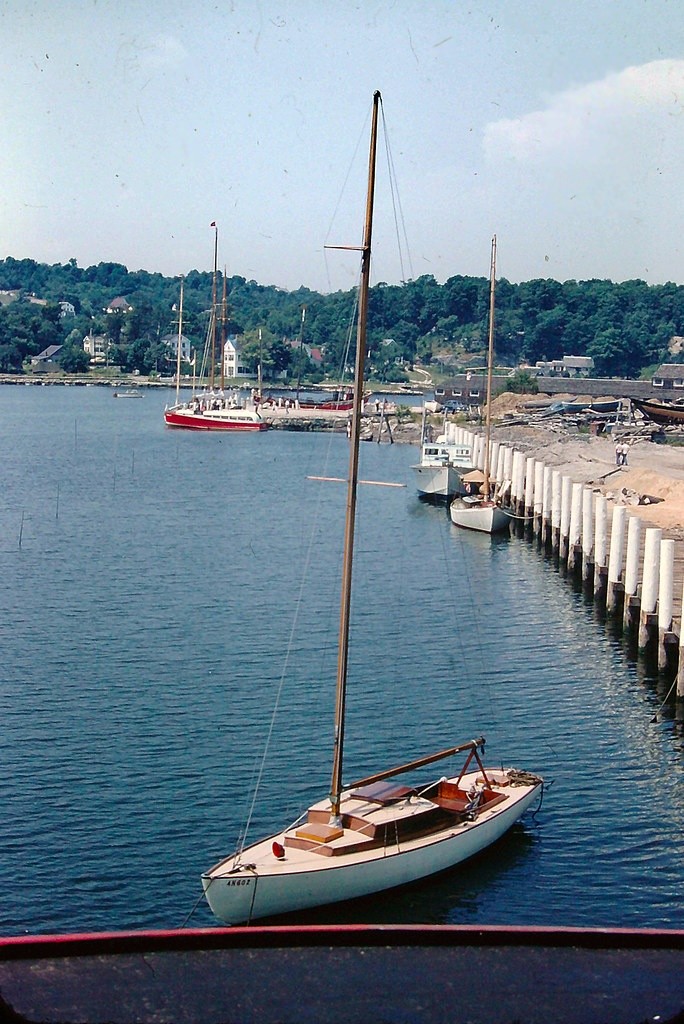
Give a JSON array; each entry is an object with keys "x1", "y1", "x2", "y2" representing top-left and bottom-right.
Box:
[
  {"x1": 616, "y1": 440, "x2": 630, "y2": 465},
  {"x1": 192, "y1": 397, "x2": 238, "y2": 415}
]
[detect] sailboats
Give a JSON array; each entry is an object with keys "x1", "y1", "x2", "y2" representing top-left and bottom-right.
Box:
[
  {"x1": 450, "y1": 232, "x2": 516, "y2": 534},
  {"x1": 162, "y1": 264, "x2": 272, "y2": 433},
  {"x1": 250, "y1": 307, "x2": 373, "y2": 411},
  {"x1": 200, "y1": 88, "x2": 554, "y2": 926}
]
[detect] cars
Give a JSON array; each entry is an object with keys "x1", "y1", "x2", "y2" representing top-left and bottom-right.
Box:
[{"x1": 443, "y1": 399, "x2": 470, "y2": 414}]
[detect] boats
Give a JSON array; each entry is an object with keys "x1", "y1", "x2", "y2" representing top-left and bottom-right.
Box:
[
  {"x1": 630, "y1": 397, "x2": 684, "y2": 426},
  {"x1": 408, "y1": 417, "x2": 485, "y2": 501},
  {"x1": 561, "y1": 397, "x2": 624, "y2": 415},
  {"x1": 113, "y1": 386, "x2": 145, "y2": 398}
]
